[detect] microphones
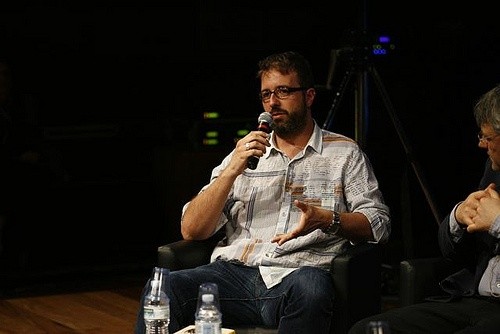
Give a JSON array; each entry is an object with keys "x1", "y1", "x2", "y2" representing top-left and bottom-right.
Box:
[{"x1": 247, "y1": 111, "x2": 274, "y2": 170}]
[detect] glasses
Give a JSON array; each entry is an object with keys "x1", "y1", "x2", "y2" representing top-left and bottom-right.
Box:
[
  {"x1": 477, "y1": 132, "x2": 500, "y2": 145},
  {"x1": 258, "y1": 85, "x2": 307, "y2": 102}
]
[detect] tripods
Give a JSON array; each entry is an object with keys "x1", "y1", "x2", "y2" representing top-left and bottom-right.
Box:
[{"x1": 321, "y1": 44, "x2": 447, "y2": 226}]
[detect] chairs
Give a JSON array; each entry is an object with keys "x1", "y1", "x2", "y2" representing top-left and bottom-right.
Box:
[{"x1": 157, "y1": 240, "x2": 381, "y2": 334}]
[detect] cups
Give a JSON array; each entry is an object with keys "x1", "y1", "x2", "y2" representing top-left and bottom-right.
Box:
[
  {"x1": 195, "y1": 283, "x2": 222, "y2": 321},
  {"x1": 142, "y1": 267, "x2": 172, "y2": 306},
  {"x1": 366, "y1": 321, "x2": 392, "y2": 334}
]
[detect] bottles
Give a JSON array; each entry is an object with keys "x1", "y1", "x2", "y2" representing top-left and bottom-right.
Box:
[
  {"x1": 195, "y1": 294, "x2": 222, "y2": 334},
  {"x1": 144, "y1": 280, "x2": 170, "y2": 334}
]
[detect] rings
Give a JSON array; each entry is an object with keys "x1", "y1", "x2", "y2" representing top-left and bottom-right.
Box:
[{"x1": 246, "y1": 143, "x2": 251, "y2": 150}]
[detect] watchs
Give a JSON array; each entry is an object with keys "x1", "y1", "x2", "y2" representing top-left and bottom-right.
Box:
[{"x1": 323, "y1": 211, "x2": 342, "y2": 236}]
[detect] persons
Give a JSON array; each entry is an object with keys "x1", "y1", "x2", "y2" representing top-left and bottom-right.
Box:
[
  {"x1": 348, "y1": 86, "x2": 500, "y2": 334},
  {"x1": 135, "y1": 51, "x2": 393, "y2": 334}
]
